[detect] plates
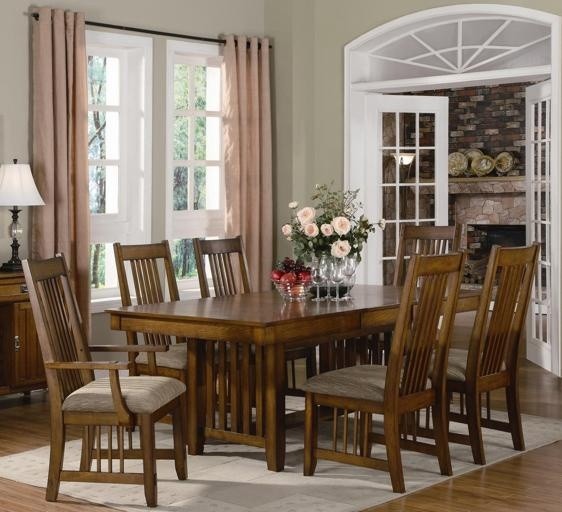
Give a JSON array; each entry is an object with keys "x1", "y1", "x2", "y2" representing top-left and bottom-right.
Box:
[
  {"x1": 471, "y1": 155, "x2": 495, "y2": 176},
  {"x1": 448, "y1": 152, "x2": 468, "y2": 176},
  {"x1": 494, "y1": 152, "x2": 513, "y2": 173},
  {"x1": 463, "y1": 149, "x2": 484, "y2": 176}
]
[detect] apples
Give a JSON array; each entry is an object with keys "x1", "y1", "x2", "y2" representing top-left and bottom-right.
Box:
[
  {"x1": 299, "y1": 271, "x2": 311, "y2": 283},
  {"x1": 282, "y1": 269, "x2": 299, "y2": 285},
  {"x1": 270, "y1": 268, "x2": 283, "y2": 281},
  {"x1": 290, "y1": 286, "x2": 304, "y2": 297}
]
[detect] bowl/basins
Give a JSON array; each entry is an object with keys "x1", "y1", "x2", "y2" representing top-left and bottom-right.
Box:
[{"x1": 271, "y1": 278, "x2": 312, "y2": 303}]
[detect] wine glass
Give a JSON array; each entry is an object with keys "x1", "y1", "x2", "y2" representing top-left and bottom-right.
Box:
[
  {"x1": 330, "y1": 258, "x2": 344, "y2": 302},
  {"x1": 318, "y1": 255, "x2": 333, "y2": 299},
  {"x1": 311, "y1": 259, "x2": 326, "y2": 301},
  {"x1": 341, "y1": 257, "x2": 357, "y2": 300}
]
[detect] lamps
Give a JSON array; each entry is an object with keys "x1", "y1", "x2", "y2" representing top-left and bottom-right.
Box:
[
  {"x1": 390, "y1": 151, "x2": 417, "y2": 226},
  {"x1": 0, "y1": 158, "x2": 46, "y2": 272}
]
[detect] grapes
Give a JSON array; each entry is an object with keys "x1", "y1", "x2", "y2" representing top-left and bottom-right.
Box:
[{"x1": 279, "y1": 256, "x2": 311, "y2": 272}]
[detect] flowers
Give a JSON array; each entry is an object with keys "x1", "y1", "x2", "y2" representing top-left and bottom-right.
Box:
[{"x1": 280, "y1": 182, "x2": 387, "y2": 266}]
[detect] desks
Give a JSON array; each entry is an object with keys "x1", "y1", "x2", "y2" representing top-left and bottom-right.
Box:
[{"x1": 0, "y1": 272, "x2": 70, "y2": 410}]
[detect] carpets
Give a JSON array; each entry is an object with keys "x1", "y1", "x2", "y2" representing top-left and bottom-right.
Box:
[{"x1": 0, "y1": 362, "x2": 562, "y2": 511}]
